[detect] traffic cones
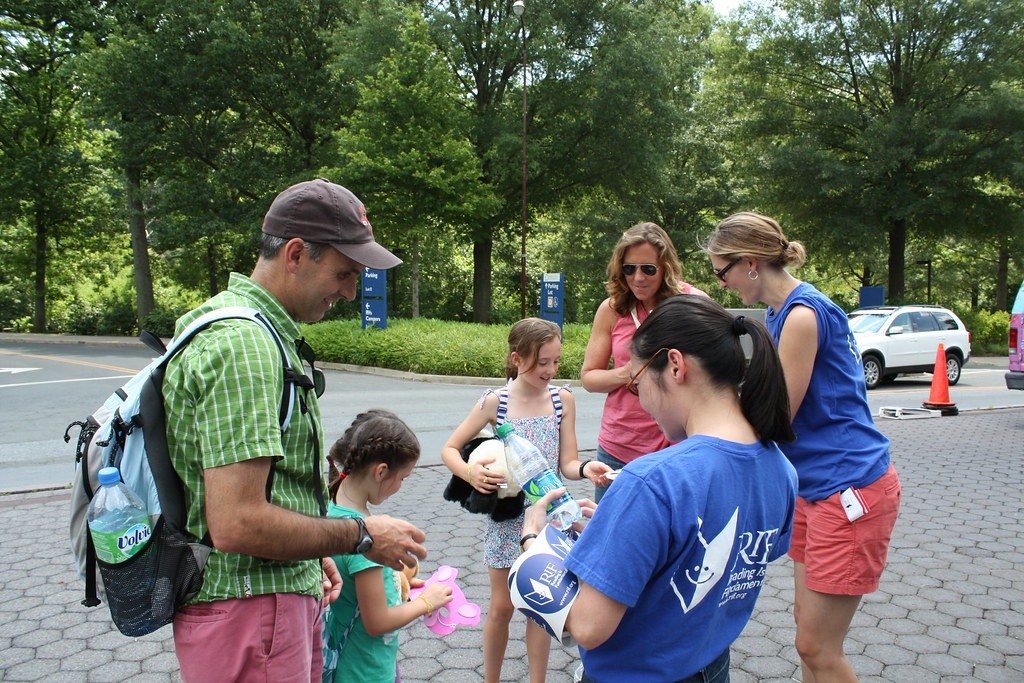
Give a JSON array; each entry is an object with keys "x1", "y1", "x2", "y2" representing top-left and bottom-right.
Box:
[{"x1": 920, "y1": 342, "x2": 959, "y2": 417}]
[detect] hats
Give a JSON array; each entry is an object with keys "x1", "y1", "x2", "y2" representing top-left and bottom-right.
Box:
[{"x1": 261, "y1": 177, "x2": 404, "y2": 270}]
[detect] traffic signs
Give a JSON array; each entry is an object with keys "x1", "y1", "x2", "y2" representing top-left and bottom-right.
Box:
[{"x1": 360, "y1": 264, "x2": 388, "y2": 330}]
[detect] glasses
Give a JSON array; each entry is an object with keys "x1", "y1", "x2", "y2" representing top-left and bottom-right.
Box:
[
  {"x1": 714, "y1": 255, "x2": 745, "y2": 282},
  {"x1": 626, "y1": 347, "x2": 673, "y2": 395},
  {"x1": 294, "y1": 335, "x2": 325, "y2": 398},
  {"x1": 622, "y1": 263, "x2": 662, "y2": 276}
]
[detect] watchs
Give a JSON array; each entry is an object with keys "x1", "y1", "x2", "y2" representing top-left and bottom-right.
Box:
[{"x1": 350, "y1": 517, "x2": 374, "y2": 555}]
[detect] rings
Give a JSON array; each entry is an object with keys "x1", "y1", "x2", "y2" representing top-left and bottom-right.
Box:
[{"x1": 484, "y1": 477, "x2": 488, "y2": 483}]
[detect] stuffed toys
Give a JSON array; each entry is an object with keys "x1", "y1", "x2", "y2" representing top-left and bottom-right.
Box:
[
  {"x1": 400, "y1": 556, "x2": 419, "y2": 602},
  {"x1": 442, "y1": 429, "x2": 524, "y2": 522}
]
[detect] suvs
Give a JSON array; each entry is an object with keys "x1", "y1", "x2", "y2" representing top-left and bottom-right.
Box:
[
  {"x1": 846, "y1": 304, "x2": 972, "y2": 391},
  {"x1": 1002, "y1": 278, "x2": 1024, "y2": 392}
]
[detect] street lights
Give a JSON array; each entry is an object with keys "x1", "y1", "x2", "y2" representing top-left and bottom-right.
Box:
[
  {"x1": 509, "y1": 0, "x2": 529, "y2": 320},
  {"x1": 915, "y1": 258, "x2": 932, "y2": 304}
]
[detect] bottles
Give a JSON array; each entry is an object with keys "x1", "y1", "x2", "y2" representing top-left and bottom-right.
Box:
[
  {"x1": 496, "y1": 421, "x2": 582, "y2": 533},
  {"x1": 89, "y1": 467, "x2": 156, "y2": 637}
]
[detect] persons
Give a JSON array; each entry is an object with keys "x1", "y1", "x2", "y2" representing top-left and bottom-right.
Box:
[
  {"x1": 326, "y1": 409, "x2": 453, "y2": 683},
  {"x1": 163, "y1": 177, "x2": 427, "y2": 683},
  {"x1": 695, "y1": 212, "x2": 900, "y2": 683},
  {"x1": 441, "y1": 318, "x2": 614, "y2": 683},
  {"x1": 582, "y1": 222, "x2": 710, "y2": 504},
  {"x1": 520, "y1": 294, "x2": 798, "y2": 683}
]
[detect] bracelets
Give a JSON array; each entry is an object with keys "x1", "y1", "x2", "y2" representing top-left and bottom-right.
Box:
[
  {"x1": 468, "y1": 466, "x2": 470, "y2": 483},
  {"x1": 520, "y1": 534, "x2": 537, "y2": 553},
  {"x1": 579, "y1": 459, "x2": 591, "y2": 478},
  {"x1": 419, "y1": 596, "x2": 433, "y2": 613}
]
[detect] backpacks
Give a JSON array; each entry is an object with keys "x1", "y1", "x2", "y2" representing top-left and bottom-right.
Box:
[{"x1": 64, "y1": 308, "x2": 319, "y2": 636}]
